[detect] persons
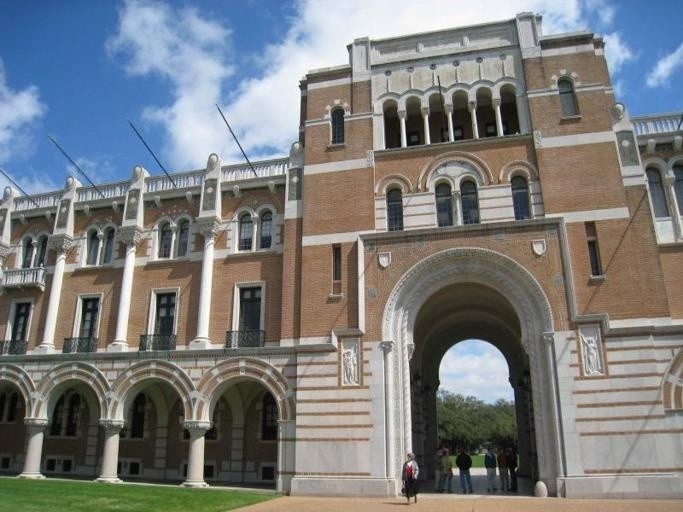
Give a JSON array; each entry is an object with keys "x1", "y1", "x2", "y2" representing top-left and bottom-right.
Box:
[
  {"x1": 401, "y1": 452, "x2": 420, "y2": 506},
  {"x1": 580, "y1": 329, "x2": 600, "y2": 375},
  {"x1": 482, "y1": 447, "x2": 498, "y2": 494},
  {"x1": 432, "y1": 449, "x2": 442, "y2": 492},
  {"x1": 436, "y1": 447, "x2": 453, "y2": 493},
  {"x1": 342, "y1": 343, "x2": 359, "y2": 386},
  {"x1": 495, "y1": 447, "x2": 510, "y2": 492},
  {"x1": 501, "y1": 446, "x2": 517, "y2": 492},
  {"x1": 453, "y1": 448, "x2": 474, "y2": 494}
]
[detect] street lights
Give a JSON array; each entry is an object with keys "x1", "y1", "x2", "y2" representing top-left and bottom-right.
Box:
[{"x1": 402, "y1": 487, "x2": 405, "y2": 493}]
